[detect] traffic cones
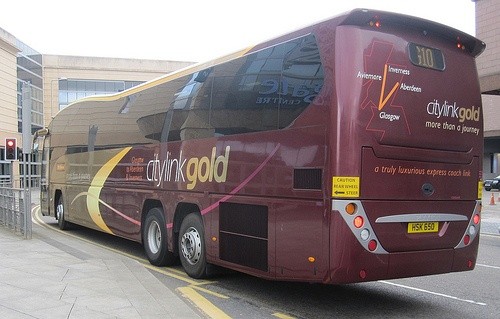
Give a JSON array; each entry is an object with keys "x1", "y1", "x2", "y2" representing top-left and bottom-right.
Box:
[{"x1": 488, "y1": 193, "x2": 496, "y2": 205}]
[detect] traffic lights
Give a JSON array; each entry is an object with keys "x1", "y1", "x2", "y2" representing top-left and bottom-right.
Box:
[{"x1": 4, "y1": 137, "x2": 18, "y2": 162}]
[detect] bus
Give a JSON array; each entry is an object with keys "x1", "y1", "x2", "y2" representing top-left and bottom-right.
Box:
[{"x1": 30, "y1": 8, "x2": 486, "y2": 285}]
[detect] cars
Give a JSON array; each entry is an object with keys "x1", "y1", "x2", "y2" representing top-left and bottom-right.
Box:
[{"x1": 483, "y1": 175, "x2": 500, "y2": 191}]
